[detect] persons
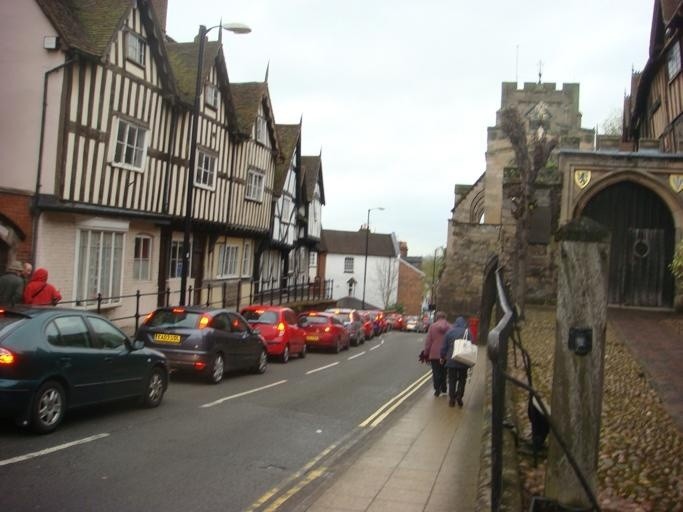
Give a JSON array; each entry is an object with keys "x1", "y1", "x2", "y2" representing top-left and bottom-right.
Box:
[
  {"x1": 23, "y1": 268, "x2": 63, "y2": 305},
  {"x1": 0, "y1": 261, "x2": 25, "y2": 306},
  {"x1": 440, "y1": 317, "x2": 472, "y2": 407},
  {"x1": 419, "y1": 311, "x2": 451, "y2": 397},
  {"x1": 20, "y1": 263, "x2": 33, "y2": 284}
]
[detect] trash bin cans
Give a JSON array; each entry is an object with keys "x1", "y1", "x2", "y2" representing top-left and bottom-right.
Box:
[{"x1": 468, "y1": 317, "x2": 480, "y2": 344}]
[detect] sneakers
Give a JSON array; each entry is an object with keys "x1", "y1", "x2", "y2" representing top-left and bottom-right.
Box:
[{"x1": 434, "y1": 387, "x2": 462, "y2": 406}]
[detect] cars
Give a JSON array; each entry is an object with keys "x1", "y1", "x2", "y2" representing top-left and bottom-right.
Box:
[
  {"x1": 0, "y1": 303, "x2": 171, "y2": 437},
  {"x1": 130, "y1": 303, "x2": 270, "y2": 387},
  {"x1": 236, "y1": 304, "x2": 310, "y2": 365},
  {"x1": 296, "y1": 306, "x2": 438, "y2": 355}
]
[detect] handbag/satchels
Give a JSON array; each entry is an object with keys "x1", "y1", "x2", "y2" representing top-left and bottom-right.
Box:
[{"x1": 450, "y1": 328, "x2": 478, "y2": 367}]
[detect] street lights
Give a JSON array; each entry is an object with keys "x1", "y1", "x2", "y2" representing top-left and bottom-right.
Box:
[
  {"x1": 177, "y1": 19, "x2": 253, "y2": 309},
  {"x1": 361, "y1": 206, "x2": 386, "y2": 310}
]
